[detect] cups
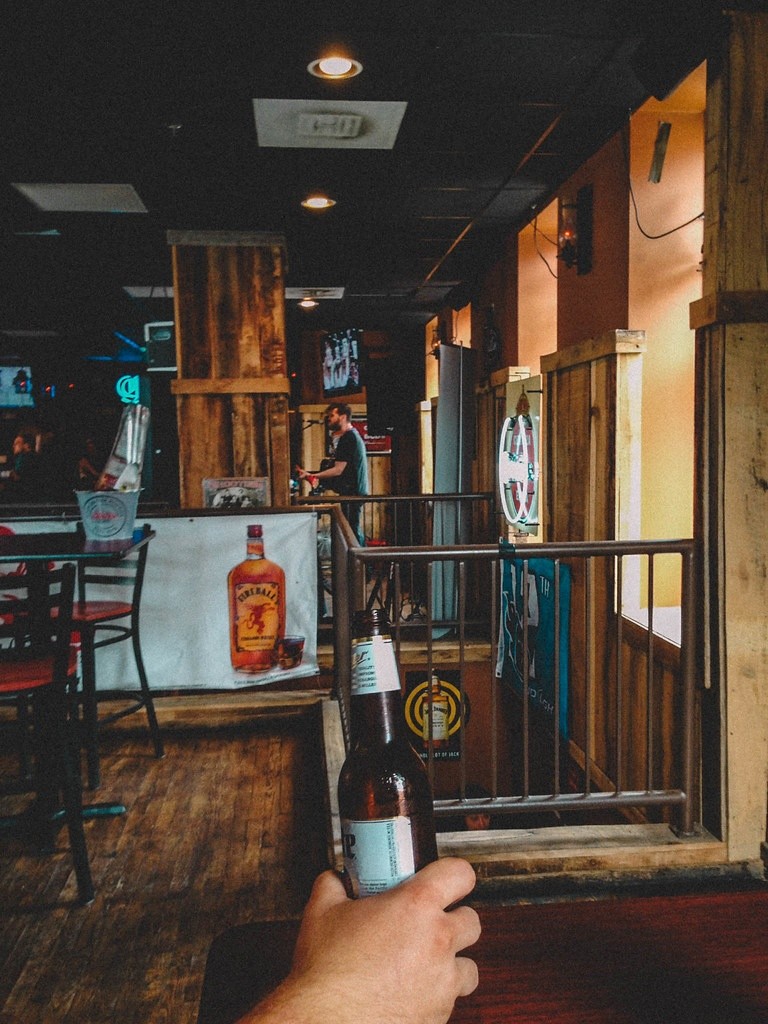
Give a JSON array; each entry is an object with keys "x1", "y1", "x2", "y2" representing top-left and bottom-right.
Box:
[{"x1": 277, "y1": 637, "x2": 305, "y2": 670}]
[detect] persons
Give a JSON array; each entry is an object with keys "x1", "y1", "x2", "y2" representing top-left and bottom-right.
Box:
[
  {"x1": 451, "y1": 784, "x2": 492, "y2": 831},
  {"x1": 323, "y1": 331, "x2": 357, "y2": 389},
  {"x1": 240, "y1": 857, "x2": 482, "y2": 1023},
  {"x1": 294, "y1": 403, "x2": 369, "y2": 543},
  {"x1": 0, "y1": 430, "x2": 100, "y2": 491},
  {"x1": 213, "y1": 489, "x2": 258, "y2": 507}
]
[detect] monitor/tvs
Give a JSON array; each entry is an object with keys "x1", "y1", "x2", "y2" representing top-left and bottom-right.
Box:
[
  {"x1": 320, "y1": 327, "x2": 363, "y2": 398},
  {"x1": 0, "y1": 362, "x2": 39, "y2": 413}
]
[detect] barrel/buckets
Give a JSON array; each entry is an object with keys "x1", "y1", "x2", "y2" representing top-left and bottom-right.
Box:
[{"x1": 73, "y1": 487, "x2": 146, "y2": 541}]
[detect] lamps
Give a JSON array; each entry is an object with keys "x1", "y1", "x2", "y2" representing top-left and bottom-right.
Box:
[{"x1": 306, "y1": 42, "x2": 365, "y2": 80}]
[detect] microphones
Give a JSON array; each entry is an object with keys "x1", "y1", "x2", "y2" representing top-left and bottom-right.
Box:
[{"x1": 307, "y1": 420, "x2": 324, "y2": 425}]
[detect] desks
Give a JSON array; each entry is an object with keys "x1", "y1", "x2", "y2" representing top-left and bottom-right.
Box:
[{"x1": 0, "y1": 529, "x2": 157, "y2": 853}]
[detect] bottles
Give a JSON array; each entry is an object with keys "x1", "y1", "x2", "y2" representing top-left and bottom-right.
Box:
[
  {"x1": 228, "y1": 524, "x2": 286, "y2": 674},
  {"x1": 95, "y1": 404, "x2": 151, "y2": 491},
  {"x1": 338, "y1": 609, "x2": 438, "y2": 901},
  {"x1": 424, "y1": 668, "x2": 450, "y2": 753}
]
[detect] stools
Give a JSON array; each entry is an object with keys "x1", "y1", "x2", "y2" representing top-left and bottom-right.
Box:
[{"x1": 0, "y1": 520, "x2": 164, "y2": 901}]
